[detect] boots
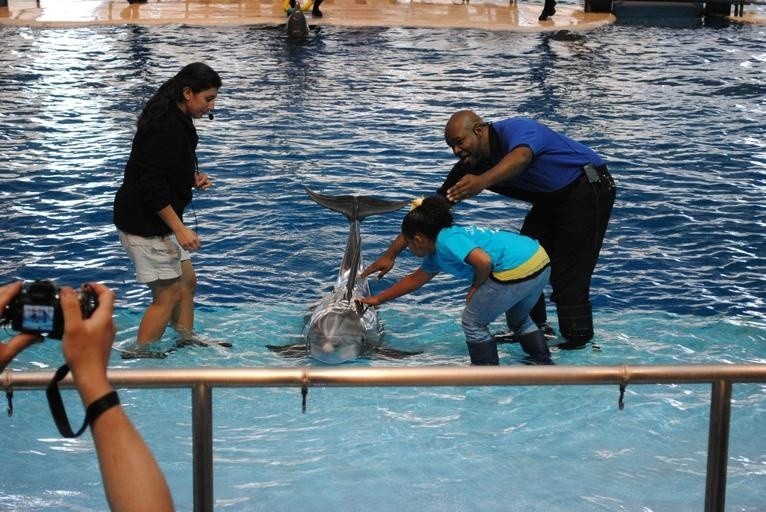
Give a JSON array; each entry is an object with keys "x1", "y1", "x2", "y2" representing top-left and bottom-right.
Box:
[{"x1": 466, "y1": 302, "x2": 593, "y2": 365}]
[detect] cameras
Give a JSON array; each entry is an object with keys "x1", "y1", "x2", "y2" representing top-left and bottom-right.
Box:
[{"x1": 9, "y1": 279, "x2": 97, "y2": 340}]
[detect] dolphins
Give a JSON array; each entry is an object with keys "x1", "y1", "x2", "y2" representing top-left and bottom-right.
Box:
[{"x1": 264, "y1": 184, "x2": 427, "y2": 365}]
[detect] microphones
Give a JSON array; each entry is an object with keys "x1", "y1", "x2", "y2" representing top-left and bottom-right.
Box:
[{"x1": 208, "y1": 109, "x2": 214, "y2": 120}]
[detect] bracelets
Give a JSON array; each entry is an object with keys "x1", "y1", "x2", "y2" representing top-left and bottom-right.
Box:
[{"x1": 471, "y1": 284, "x2": 478, "y2": 288}]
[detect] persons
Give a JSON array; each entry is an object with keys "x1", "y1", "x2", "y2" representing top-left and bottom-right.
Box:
[
  {"x1": 354, "y1": 194, "x2": 554, "y2": 365},
  {"x1": 112, "y1": 62, "x2": 222, "y2": 343},
  {"x1": 283, "y1": 0, "x2": 323, "y2": 16},
  {"x1": 0, "y1": 277, "x2": 176, "y2": 512},
  {"x1": 358, "y1": 110, "x2": 615, "y2": 349},
  {"x1": 539, "y1": 0, "x2": 556, "y2": 20}
]
